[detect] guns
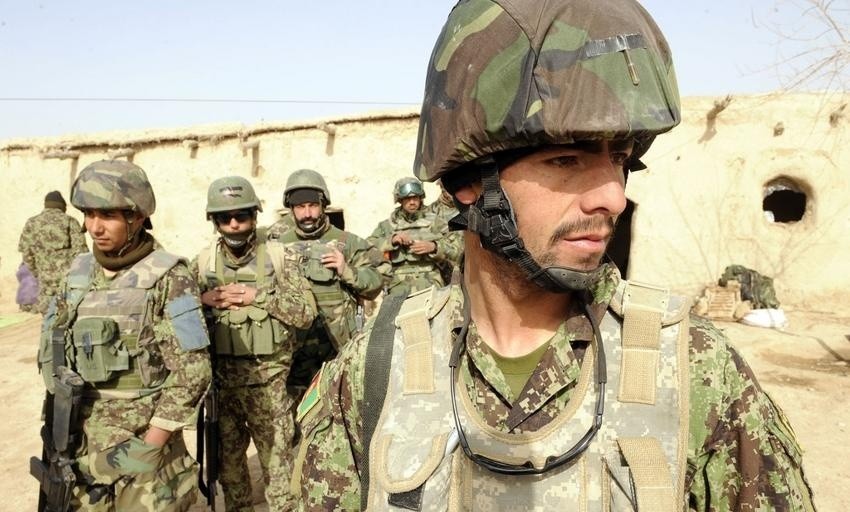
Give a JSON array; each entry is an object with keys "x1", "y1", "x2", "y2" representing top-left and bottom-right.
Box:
[
  {"x1": 30, "y1": 365, "x2": 87, "y2": 511},
  {"x1": 203, "y1": 312, "x2": 223, "y2": 512}
]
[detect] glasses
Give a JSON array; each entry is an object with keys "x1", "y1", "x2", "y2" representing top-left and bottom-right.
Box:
[
  {"x1": 216, "y1": 212, "x2": 253, "y2": 224},
  {"x1": 396, "y1": 182, "x2": 424, "y2": 195}
]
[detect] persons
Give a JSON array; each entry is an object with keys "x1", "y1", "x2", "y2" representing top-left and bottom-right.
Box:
[
  {"x1": 38, "y1": 159, "x2": 215, "y2": 512},
  {"x1": 263, "y1": 0, "x2": 819, "y2": 512},
  {"x1": 17, "y1": 191, "x2": 90, "y2": 317},
  {"x1": 187, "y1": 176, "x2": 318, "y2": 512},
  {"x1": 426, "y1": 179, "x2": 465, "y2": 285},
  {"x1": 266, "y1": 169, "x2": 394, "y2": 448},
  {"x1": 365, "y1": 177, "x2": 463, "y2": 301}
]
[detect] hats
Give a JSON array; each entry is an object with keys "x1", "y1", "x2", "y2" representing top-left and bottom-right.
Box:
[
  {"x1": 286, "y1": 188, "x2": 326, "y2": 206},
  {"x1": 45, "y1": 192, "x2": 66, "y2": 205}
]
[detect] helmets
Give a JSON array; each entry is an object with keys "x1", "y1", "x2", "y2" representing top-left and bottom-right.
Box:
[
  {"x1": 413, "y1": 0, "x2": 682, "y2": 181},
  {"x1": 71, "y1": 160, "x2": 155, "y2": 217},
  {"x1": 206, "y1": 177, "x2": 263, "y2": 221},
  {"x1": 394, "y1": 177, "x2": 426, "y2": 203},
  {"x1": 282, "y1": 169, "x2": 331, "y2": 208}
]
